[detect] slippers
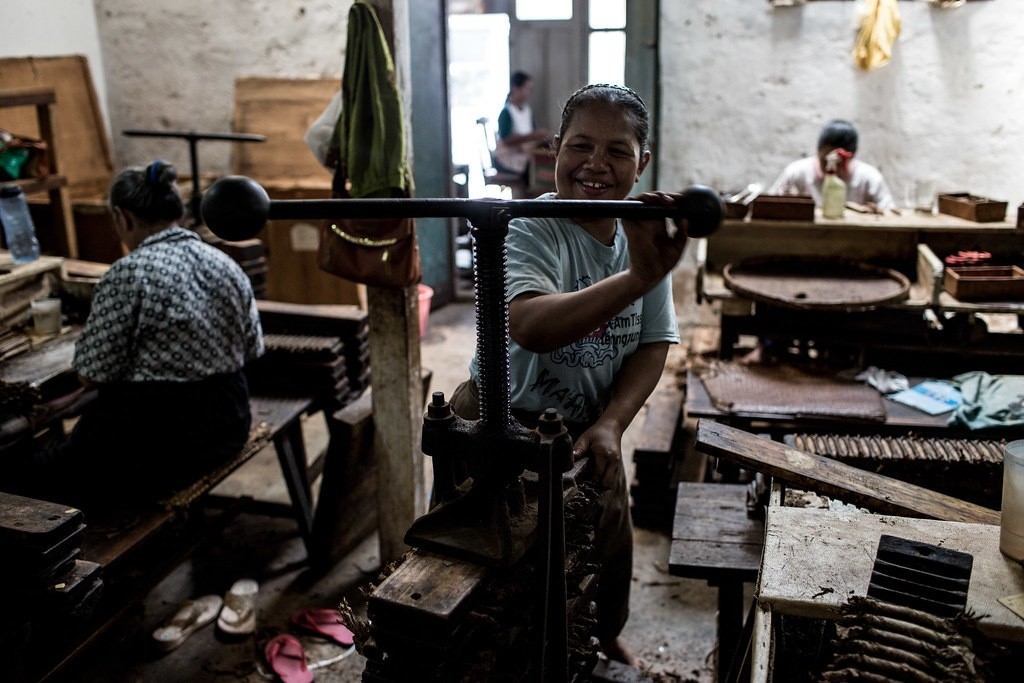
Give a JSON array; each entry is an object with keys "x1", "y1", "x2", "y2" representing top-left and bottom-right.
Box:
[
  {"x1": 265, "y1": 633, "x2": 314, "y2": 683},
  {"x1": 291, "y1": 608, "x2": 355, "y2": 646},
  {"x1": 217, "y1": 578, "x2": 258, "y2": 634},
  {"x1": 152, "y1": 594, "x2": 223, "y2": 651}
]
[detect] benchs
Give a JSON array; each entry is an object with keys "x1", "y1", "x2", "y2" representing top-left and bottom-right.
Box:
[
  {"x1": 0, "y1": 394, "x2": 317, "y2": 683},
  {"x1": 484, "y1": 166, "x2": 527, "y2": 200},
  {"x1": 628, "y1": 390, "x2": 686, "y2": 527}
]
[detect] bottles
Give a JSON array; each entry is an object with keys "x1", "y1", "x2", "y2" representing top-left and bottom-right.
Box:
[{"x1": 0, "y1": 185, "x2": 40, "y2": 263}]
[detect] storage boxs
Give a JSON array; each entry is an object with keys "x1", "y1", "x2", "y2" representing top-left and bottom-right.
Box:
[
  {"x1": 943, "y1": 266, "x2": 1024, "y2": 303},
  {"x1": 937, "y1": 191, "x2": 1009, "y2": 224}
]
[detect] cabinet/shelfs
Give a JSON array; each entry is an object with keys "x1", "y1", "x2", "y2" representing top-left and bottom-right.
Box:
[{"x1": 255, "y1": 186, "x2": 366, "y2": 313}]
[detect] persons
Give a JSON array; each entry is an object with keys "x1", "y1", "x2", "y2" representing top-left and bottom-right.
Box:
[
  {"x1": 770, "y1": 120, "x2": 894, "y2": 209},
  {"x1": 61, "y1": 161, "x2": 262, "y2": 533},
  {"x1": 427, "y1": 84, "x2": 680, "y2": 665},
  {"x1": 494, "y1": 71, "x2": 550, "y2": 189}
]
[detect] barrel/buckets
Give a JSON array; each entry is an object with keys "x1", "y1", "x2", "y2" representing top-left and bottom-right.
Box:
[{"x1": 418, "y1": 284, "x2": 433, "y2": 341}]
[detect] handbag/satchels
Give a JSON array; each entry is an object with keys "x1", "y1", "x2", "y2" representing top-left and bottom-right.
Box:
[
  {"x1": 305, "y1": 88, "x2": 344, "y2": 175},
  {"x1": 317, "y1": 190, "x2": 422, "y2": 288}
]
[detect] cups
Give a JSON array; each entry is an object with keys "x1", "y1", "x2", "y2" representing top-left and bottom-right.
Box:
[
  {"x1": 31, "y1": 298, "x2": 61, "y2": 336},
  {"x1": 999, "y1": 440, "x2": 1024, "y2": 562}
]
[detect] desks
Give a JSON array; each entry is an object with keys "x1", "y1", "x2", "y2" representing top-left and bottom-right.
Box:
[
  {"x1": 695, "y1": 207, "x2": 1024, "y2": 369},
  {"x1": 667, "y1": 481, "x2": 764, "y2": 683}
]
